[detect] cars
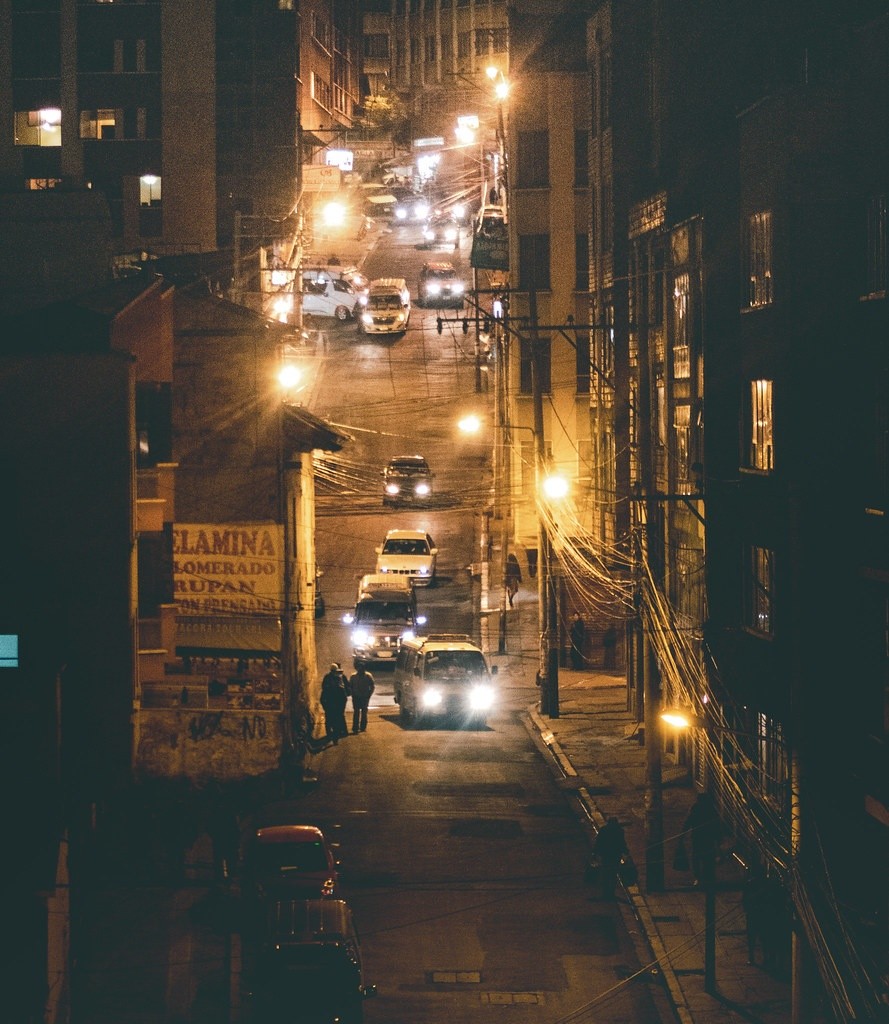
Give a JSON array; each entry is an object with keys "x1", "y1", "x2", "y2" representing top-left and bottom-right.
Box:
[
  {"x1": 380, "y1": 455, "x2": 438, "y2": 510},
  {"x1": 242, "y1": 824, "x2": 340, "y2": 912},
  {"x1": 374, "y1": 529, "x2": 439, "y2": 588}
]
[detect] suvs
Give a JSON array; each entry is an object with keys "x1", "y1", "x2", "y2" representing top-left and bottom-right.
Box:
[
  {"x1": 419, "y1": 261, "x2": 465, "y2": 308},
  {"x1": 360, "y1": 276, "x2": 411, "y2": 336}
]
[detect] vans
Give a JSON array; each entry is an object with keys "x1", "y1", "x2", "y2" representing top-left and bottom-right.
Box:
[
  {"x1": 300, "y1": 265, "x2": 369, "y2": 322},
  {"x1": 348, "y1": 572, "x2": 421, "y2": 673},
  {"x1": 253, "y1": 895, "x2": 377, "y2": 1024},
  {"x1": 396, "y1": 633, "x2": 498, "y2": 730}
]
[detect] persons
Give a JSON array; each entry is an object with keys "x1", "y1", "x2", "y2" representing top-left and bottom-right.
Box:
[
  {"x1": 528, "y1": 548, "x2": 538, "y2": 578},
  {"x1": 604, "y1": 624, "x2": 617, "y2": 666},
  {"x1": 680, "y1": 792, "x2": 719, "y2": 887},
  {"x1": 320, "y1": 662, "x2": 376, "y2": 737},
  {"x1": 593, "y1": 818, "x2": 629, "y2": 890},
  {"x1": 569, "y1": 614, "x2": 585, "y2": 664},
  {"x1": 504, "y1": 553, "x2": 522, "y2": 607}
]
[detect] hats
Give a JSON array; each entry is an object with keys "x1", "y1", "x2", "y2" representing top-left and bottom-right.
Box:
[{"x1": 330, "y1": 664, "x2": 338, "y2": 670}]
